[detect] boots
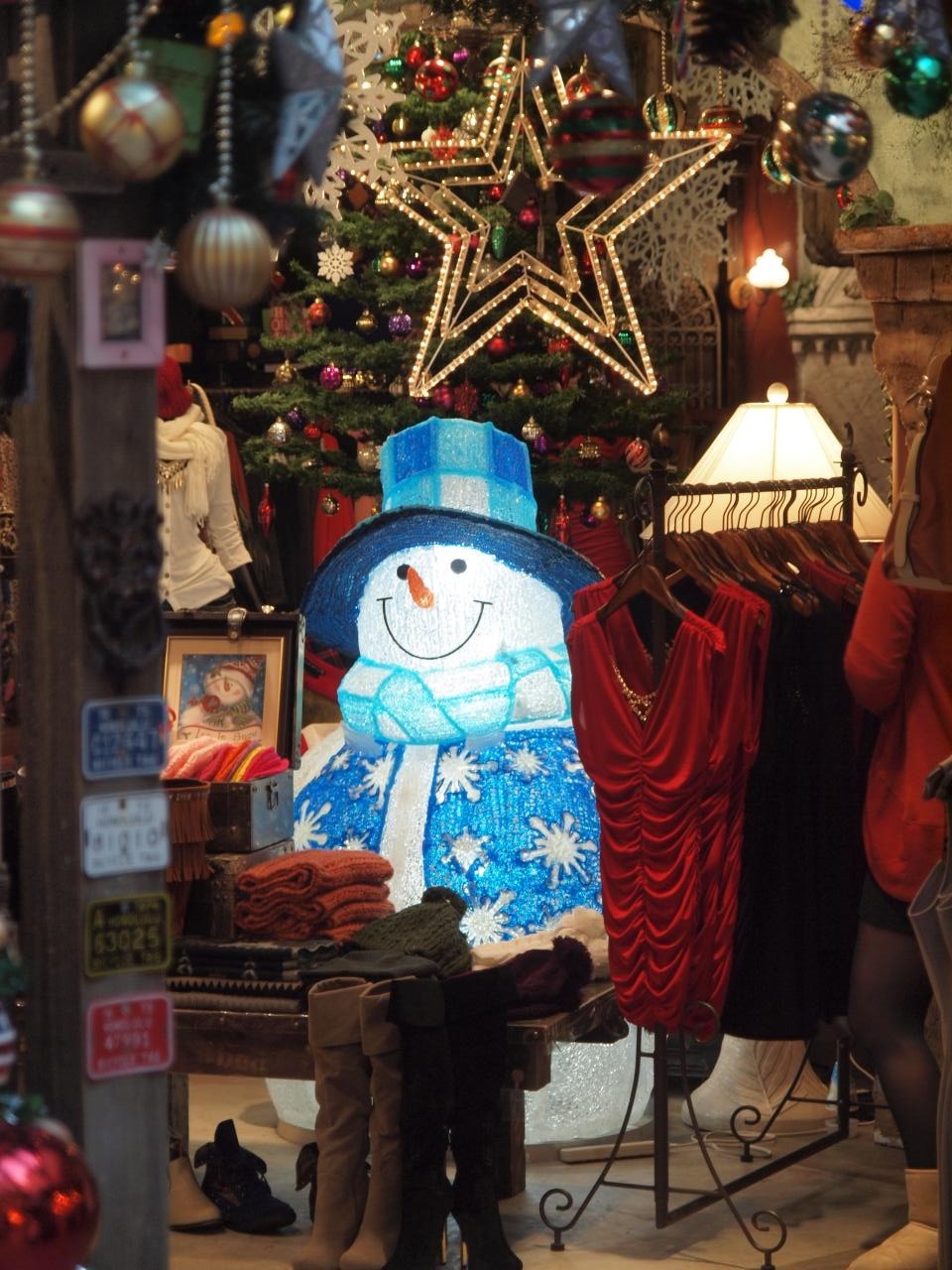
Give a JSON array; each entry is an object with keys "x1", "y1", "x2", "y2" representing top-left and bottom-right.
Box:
[
  {"x1": 449, "y1": 967, "x2": 524, "y2": 1270},
  {"x1": 292, "y1": 977, "x2": 372, "y2": 1270},
  {"x1": 341, "y1": 977, "x2": 453, "y2": 1270},
  {"x1": 846, "y1": 1171, "x2": 945, "y2": 1270}
]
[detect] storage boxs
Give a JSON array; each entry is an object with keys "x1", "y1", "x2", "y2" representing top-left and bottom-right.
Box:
[{"x1": 190, "y1": 770, "x2": 297, "y2": 943}]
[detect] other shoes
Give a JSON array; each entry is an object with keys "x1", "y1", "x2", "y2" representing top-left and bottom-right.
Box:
[
  {"x1": 168, "y1": 1126, "x2": 222, "y2": 1230},
  {"x1": 295, "y1": 1142, "x2": 318, "y2": 1223},
  {"x1": 193, "y1": 1119, "x2": 298, "y2": 1232},
  {"x1": 825, "y1": 1102, "x2": 876, "y2": 1126}
]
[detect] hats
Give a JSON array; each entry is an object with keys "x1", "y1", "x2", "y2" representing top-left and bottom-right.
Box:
[
  {"x1": 345, "y1": 886, "x2": 472, "y2": 994},
  {"x1": 156, "y1": 356, "x2": 193, "y2": 419},
  {"x1": 455, "y1": 936, "x2": 593, "y2": 1019},
  {"x1": 471, "y1": 907, "x2": 609, "y2": 982}
]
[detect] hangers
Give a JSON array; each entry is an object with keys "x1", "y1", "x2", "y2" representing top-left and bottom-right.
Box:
[{"x1": 569, "y1": 477, "x2": 877, "y2": 654}]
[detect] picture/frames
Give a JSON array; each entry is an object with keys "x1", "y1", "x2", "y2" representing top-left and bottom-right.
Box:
[
  {"x1": 159, "y1": 605, "x2": 306, "y2": 773},
  {"x1": 73, "y1": 239, "x2": 167, "y2": 373}
]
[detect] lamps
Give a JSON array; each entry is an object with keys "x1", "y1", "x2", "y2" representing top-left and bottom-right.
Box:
[
  {"x1": 639, "y1": 384, "x2": 895, "y2": 1134},
  {"x1": 729, "y1": 248, "x2": 789, "y2": 313}
]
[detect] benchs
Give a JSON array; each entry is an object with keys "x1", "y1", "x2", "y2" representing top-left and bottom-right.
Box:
[{"x1": 173, "y1": 983, "x2": 620, "y2": 1201}]
